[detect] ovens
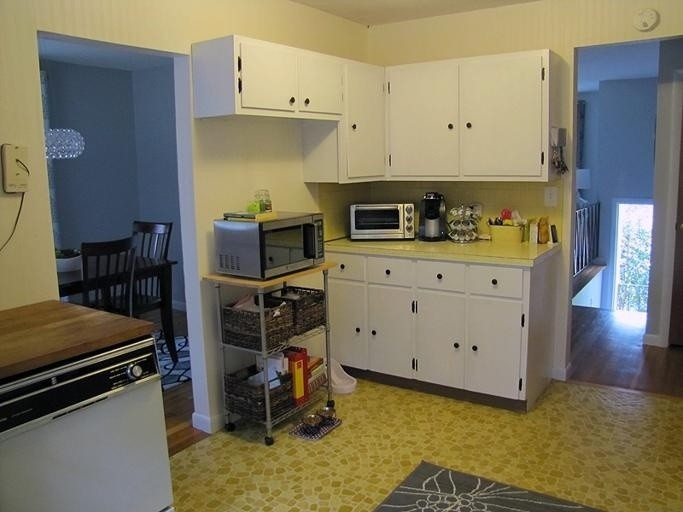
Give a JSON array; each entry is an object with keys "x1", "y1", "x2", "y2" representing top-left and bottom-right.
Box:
[{"x1": 347, "y1": 201, "x2": 415, "y2": 242}]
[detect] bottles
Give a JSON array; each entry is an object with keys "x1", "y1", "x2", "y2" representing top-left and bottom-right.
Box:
[{"x1": 246, "y1": 189, "x2": 272, "y2": 215}]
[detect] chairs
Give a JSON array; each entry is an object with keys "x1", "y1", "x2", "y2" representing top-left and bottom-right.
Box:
[
  {"x1": 79, "y1": 232, "x2": 138, "y2": 318},
  {"x1": 88, "y1": 217, "x2": 172, "y2": 319}
]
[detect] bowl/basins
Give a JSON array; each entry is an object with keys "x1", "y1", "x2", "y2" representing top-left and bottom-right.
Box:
[
  {"x1": 301, "y1": 413, "x2": 323, "y2": 429},
  {"x1": 319, "y1": 407, "x2": 336, "y2": 421},
  {"x1": 488, "y1": 226, "x2": 522, "y2": 244},
  {"x1": 55, "y1": 255, "x2": 81, "y2": 272}
]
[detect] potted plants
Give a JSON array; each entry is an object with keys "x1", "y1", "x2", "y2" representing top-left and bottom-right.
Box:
[{"x1": 54, "y1": 247, "x2": 81, "y2": 273}]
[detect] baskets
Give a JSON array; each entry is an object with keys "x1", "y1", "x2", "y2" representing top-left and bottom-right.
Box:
[
  {"x1": 225, "y1": 364, "x2": 294, "y2": 422},
  {"x1": 221, "y1": 286, "x2": 326, "y2": 353}
]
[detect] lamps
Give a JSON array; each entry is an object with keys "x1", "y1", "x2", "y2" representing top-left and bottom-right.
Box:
[
  {"x1": 42, "y1": 128, "x2": 86, "y2": 159},
  {"x1": 575, "y1": 169, "x2": 592, "y2": 208}
]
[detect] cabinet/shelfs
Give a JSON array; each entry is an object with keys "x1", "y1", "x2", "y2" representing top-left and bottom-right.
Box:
[
  {"x1": 384, "y1": 46, "x2": 561, "y2": 188},
  {"x1": 201, "y1": 261, "x2": 338, "y2": 445},
  {"x1": 187, "y1": 35, "x2": 343, "y2": 127},
  {"x1": 320, "y1": 251, "x2": 415, "y2": 382},
  {"x1": 300, "y1": 57, "x2": 384, "y2": 186},
  {"x1": 412, "y1": 254, "x2": 556, "y2": 403}
]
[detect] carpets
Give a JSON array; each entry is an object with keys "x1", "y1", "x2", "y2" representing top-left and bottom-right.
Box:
[
  {"x1": 370, "y1": 455, "x2": 602, "y2": 510},
  {"x1": 149, "y1": 329, "x2": 191, "y2": 394}
]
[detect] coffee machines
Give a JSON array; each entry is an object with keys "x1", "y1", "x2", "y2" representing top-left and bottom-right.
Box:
[{"x1": 417, "y1": 192, "x2": 449, "y2": 240}]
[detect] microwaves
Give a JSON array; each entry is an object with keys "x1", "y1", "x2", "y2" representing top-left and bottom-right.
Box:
[{"x1": 213, "y1": 211, "x2": 324, "y2": 281}]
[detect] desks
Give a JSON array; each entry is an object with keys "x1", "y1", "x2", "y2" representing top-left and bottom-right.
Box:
[{"x1": 54, "y1": 254, "x2": 178, "y2": 364}]
